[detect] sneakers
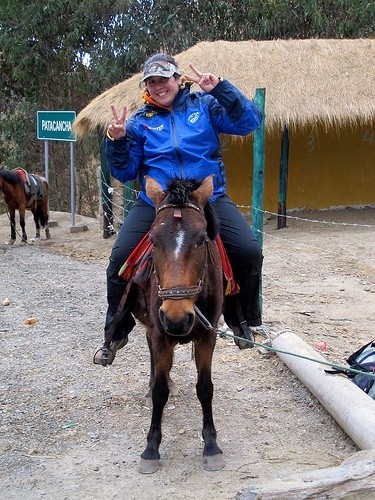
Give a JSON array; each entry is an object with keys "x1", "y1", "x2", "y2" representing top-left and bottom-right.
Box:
[
  {"x1": 228, "y1": 322, "x2": 255, "y2": 349},
  {"x1": 96, "y1": 337, "x2": 129, "y2": 365}
]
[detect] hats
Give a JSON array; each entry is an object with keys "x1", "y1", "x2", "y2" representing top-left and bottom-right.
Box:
[{"x1": 141, "y1": 54, "x2": 180, "y2": 83}]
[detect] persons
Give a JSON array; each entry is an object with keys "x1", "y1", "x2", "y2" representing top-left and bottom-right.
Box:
[{"x1": 92, "y1": 54, "x2": 266, "y2": 366}]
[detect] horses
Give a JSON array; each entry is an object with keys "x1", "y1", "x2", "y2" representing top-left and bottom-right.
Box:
[
  {"x1": 0, "y1": 167, "x2": 53, "y2": 246},
  {"x1": 126, "y1": 173, "x2": 226, "y2": 474}
]
